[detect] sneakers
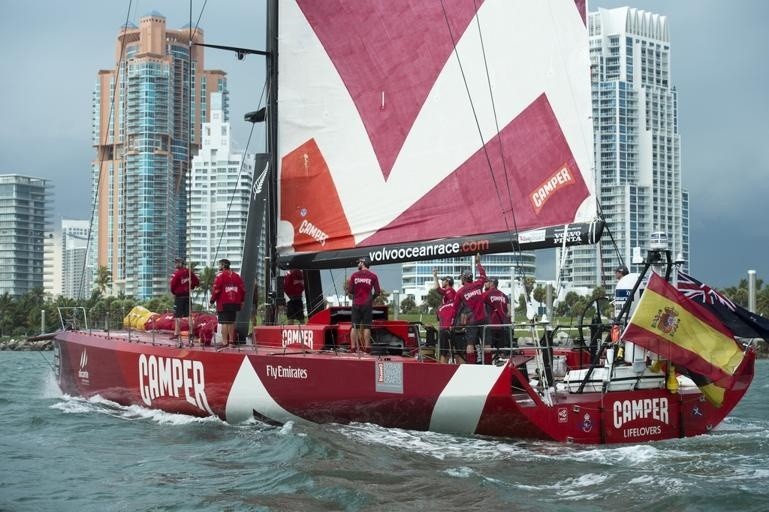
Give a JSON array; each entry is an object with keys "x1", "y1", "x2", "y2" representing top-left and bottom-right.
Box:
[
  {"x1": 169, "y1": 334, "x2": 179, "y2": 341},
  {"x1": 217, "y1": 340, "x2": 237, "y2": 349}
]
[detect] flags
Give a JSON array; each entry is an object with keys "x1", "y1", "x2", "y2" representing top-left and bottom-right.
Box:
[
  {"x1": 622, "y1": 272, "x2": 747, "y2": 391},
  {"x1": 677, "y1": 269, "x2": 768, "y2": 345}
]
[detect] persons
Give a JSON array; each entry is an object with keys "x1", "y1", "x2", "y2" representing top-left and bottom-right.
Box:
[
  {"x1": 208, "y1": 259, "x2": 247, "y2": 348},
  {"x1": 347, "y1": 257, "x2": 381, "y2": 353},
  {"x1": 282, "y1": 268, "x2": 305, "y2": 325},
  {"x1": 250, "y1": 278, "x2": 258, "y2": 336},
  {"x1": 169, "y1": 258, "x2": 200, "y2": 340},
  {"x1": 433, "y1": 251, "x2": 514, "y2": 364},
  {"x1": 614, "y1": 266, "x2": 629, "y2": 366}
]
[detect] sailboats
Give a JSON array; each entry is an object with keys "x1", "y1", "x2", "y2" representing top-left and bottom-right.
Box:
[{"x1": 27, "y1": 0, "x2": 760, "y2": 451}]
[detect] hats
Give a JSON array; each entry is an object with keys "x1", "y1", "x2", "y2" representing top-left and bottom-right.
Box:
[
  {"x1": 355, "y1": 256, "x2": 372, "y2": 266},
  {"x1": 613, "y1": 265, "x2": 629, "y2": 272},
  {"x1": 440, "y1": 275, "x2": 455, "y2": 286},
  {"x1": 459, "y1": 270, "x2": 473, "y2": 281},
  {"x1": 484, "y1": 276, "x2": 498, "y2": 283},
  {"x1": 173, "y1": 256, "x2": 185, "y2": 265}
]
